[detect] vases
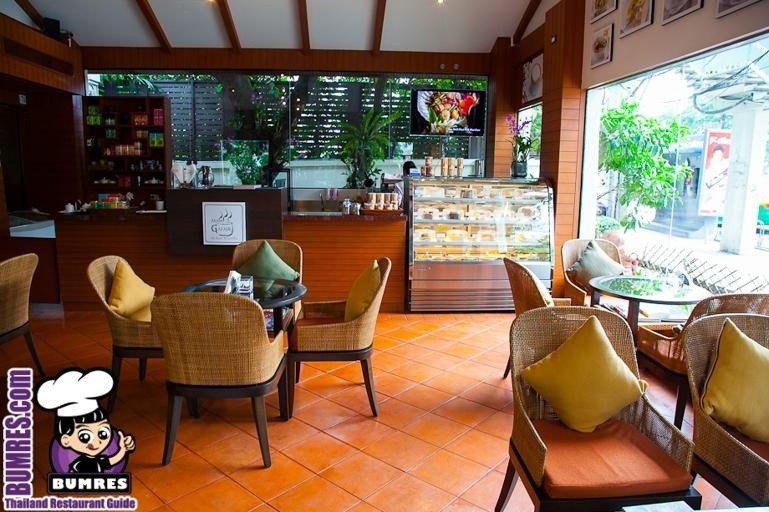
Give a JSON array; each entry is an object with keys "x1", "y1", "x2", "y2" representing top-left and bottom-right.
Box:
[{"x1": 510, "y1": 161, "x2": 520, "y2": 177}]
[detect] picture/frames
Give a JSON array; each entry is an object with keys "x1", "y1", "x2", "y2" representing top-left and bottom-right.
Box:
[
  {"x1": 589, "y1": 23, "x2": 613, "y2": 69},
  {"x1": 662, "y1": 0, "x2": 703, "y2": 25},
  {"x1": 618, "y1": 1, "x2": 652, "y2": 39},
  {"x1": 588, "y1": 0, "x2": 617, "y2": 26},
  {"x1": 714, "y1": 0, "x2": 760, "y2": 20}
]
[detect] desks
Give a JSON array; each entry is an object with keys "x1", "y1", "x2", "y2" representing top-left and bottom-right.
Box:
[
  {"x1": 586, "y1": 273, "x2": 713, "y2": 346},
  {"x1": 178, "y1": 277, "x2": 307, "y2": 411}
]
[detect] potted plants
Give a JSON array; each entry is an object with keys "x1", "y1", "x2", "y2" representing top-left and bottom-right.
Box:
[{"x1": 511, "y1": 134, "x2": 538, "y2": 177}]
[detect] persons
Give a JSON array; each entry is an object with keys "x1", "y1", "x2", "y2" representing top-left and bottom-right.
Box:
[{"x1": 393, "y1": 161, "x2": 417, "y2": 207}]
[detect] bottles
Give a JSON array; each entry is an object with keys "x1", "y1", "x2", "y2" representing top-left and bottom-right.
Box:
[
  {"x1": 421, "y1": 156, "x2": 463, "y2": 177},
  {"x1": 342, "y1": 198, "x2": 352, "y2": 216},
  {"x1": 364, "y1": 193, "x2": 398, "y2": 210}
]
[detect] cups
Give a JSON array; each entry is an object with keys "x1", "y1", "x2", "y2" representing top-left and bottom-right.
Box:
[
  {"x1": 155, "y1": 200, "x2": 164, "y2": 210},
  {"x1": 65, "y1": 202, "x2": 74, "y2": 212}
]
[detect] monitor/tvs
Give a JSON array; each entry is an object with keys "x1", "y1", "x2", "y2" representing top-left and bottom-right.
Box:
[{"x1": 410, "y1": 89, "x2": 486, "y2": 136}]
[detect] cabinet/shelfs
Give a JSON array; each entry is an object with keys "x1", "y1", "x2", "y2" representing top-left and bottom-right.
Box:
[
  {"x1": 81, "y1": 94, "x2": 172, "y2": 210},
  {"x1": 406, "y1": 176, "x2": 557, "y2": 314}
]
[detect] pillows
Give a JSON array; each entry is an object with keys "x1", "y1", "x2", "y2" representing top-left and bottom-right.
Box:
[
  {"x1": 701, "y1": 315, "x2": 768, "y2": 444},
  {"x1": 235, "y1": 242, "x2": 301, "y2": 299},
  {"x1": 519, "y1": 316, "x2": 648, "y2": 433},
  {"x1": 671, "y1": 322, "x2": 686, "y2": 335},
  {"x1": 106, "y1": 261, "x2": 155, "y2": 315},
  {"x1": 343, "y1": 259, "x2": 381, "y2": 322},
  {"x1": 566, "y1": 238, "x2": 626, "y2": 294}
]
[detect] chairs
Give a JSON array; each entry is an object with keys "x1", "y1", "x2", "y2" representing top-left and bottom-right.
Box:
[
  {"x1": 685, "y1": 311, "x2": 769, "y2": 507},
  {"x1": 493, "y1": 305, "x2": 702, "y2": 512},
  {"x1": 636, "y1": 293, "x2": 768, "y2": 454},
  {"x1": 231, "y1": 237, "x2": 305, "y2": 282},
  {"x1": 295, "y1": 257, "x2": 393, "y2": 418},
  {"x1": 498, "y1": 257, "x2": 572, "y2": 379},
  {"x1": 148, "y1": 291, "x2": 290, "y2": 468},
  {"x1": 561, "y1": 239, "x2": 636, "y2": 309},
  {"x1": 0, "y1": 253, "x2": 47, "y2": 385},
  {"x1": 86, "y1": 254, "x2": 163, "y2": 418}
]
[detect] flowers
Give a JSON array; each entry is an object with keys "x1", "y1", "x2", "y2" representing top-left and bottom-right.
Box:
[{"x1": 503, "y1": 114, "x2": 532, "y2": 161}]
